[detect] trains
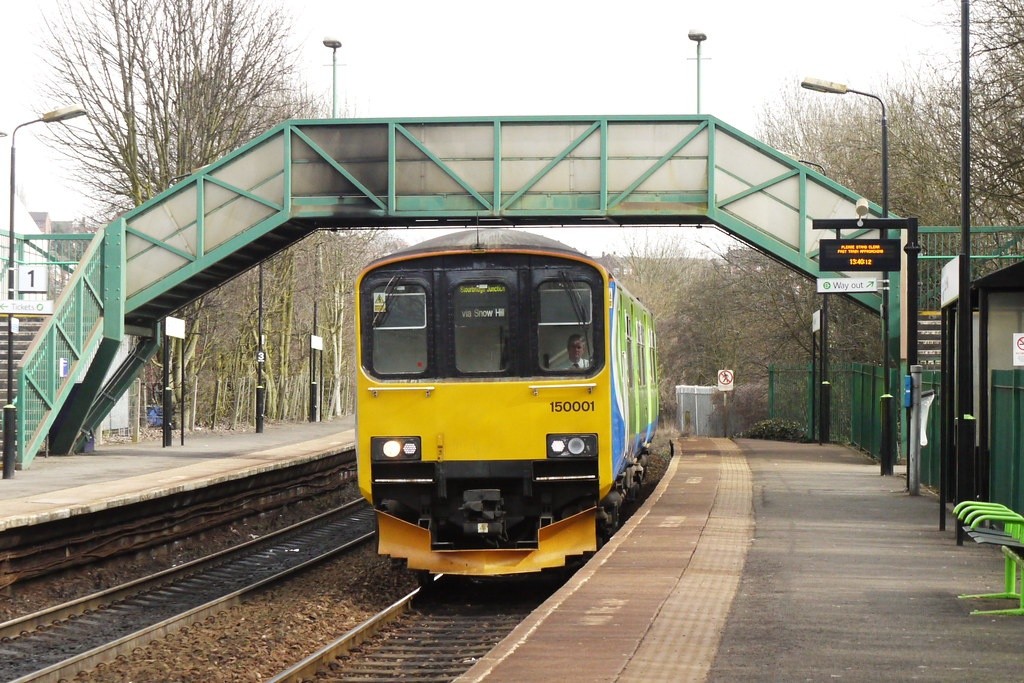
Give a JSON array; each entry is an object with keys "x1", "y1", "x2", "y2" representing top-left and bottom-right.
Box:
[{"x1": 352, "y1": 212, "x2": 665, "y2": 580}]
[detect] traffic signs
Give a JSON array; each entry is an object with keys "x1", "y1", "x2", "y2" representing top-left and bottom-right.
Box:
[{"x1": 817, "y1": 277, "x2": 879, "y2": 293}]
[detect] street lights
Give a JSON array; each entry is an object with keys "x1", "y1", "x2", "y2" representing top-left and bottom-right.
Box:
[
  {"x1": 800, "y1": 77, "x2": 902, "y2": 481},
  {"x1": 687, "y1": 31, "x2": 713, "y2": 114},
  {"x1": 321, "y1": 39, "x2": 342, "y2": 117},
  {"x1": 4, "y1": 103, "x2": 92, "y2": 482}
]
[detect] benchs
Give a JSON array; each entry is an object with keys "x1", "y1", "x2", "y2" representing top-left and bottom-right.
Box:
[{"x1": 955, "y1": 500, "x2": 1024, "y2": 617}]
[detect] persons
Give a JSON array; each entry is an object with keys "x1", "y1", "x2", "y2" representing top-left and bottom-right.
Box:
[{"x1": 552, "y1": 333, "x2": 593, "y2": 373}]
